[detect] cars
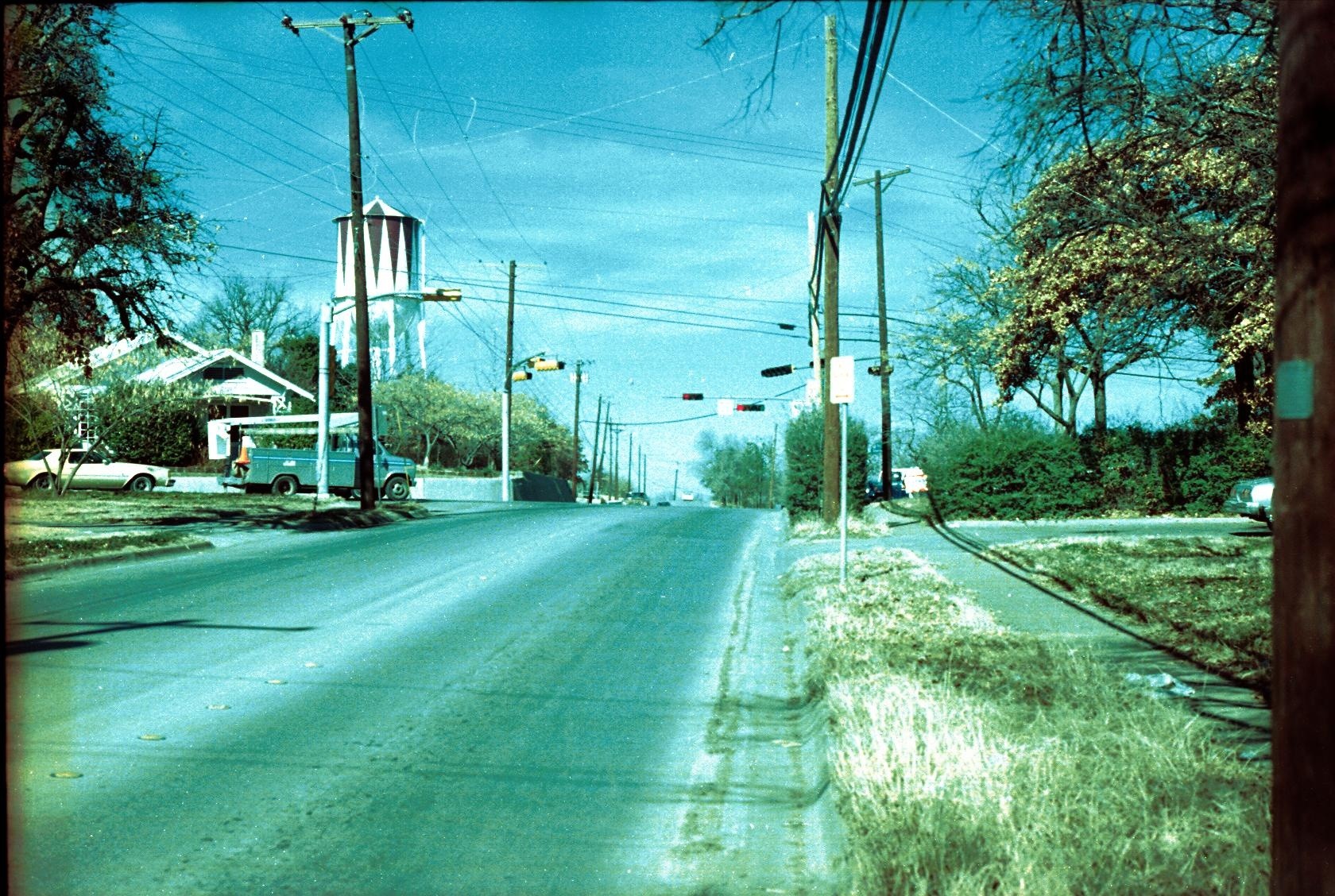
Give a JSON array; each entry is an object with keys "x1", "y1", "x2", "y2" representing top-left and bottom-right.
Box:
[{"x1": 3, "y1": 446, "x2": 171, "y2": 492}]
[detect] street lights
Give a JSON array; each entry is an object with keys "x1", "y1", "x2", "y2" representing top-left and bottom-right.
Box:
[{"x1": 311, "y1": 284, "x2": 465, "y2": 510}]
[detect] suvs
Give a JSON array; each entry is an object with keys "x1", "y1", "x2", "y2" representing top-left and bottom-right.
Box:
[
  {"x1": 1227, "y1": 475, "x2": 1277, "y2": 530},
  {"x1": 621, "y1": 492, "x2": 650, "y2": 507}
]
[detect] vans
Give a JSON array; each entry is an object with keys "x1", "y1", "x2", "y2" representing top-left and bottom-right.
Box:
[{"x1": 876, "y1": 466, "x2": 928, "y2": 494}]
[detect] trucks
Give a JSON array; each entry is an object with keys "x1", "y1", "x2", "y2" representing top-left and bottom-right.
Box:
[{"x1": 218, "y1": 431, "x2": 418, "y2": 502}]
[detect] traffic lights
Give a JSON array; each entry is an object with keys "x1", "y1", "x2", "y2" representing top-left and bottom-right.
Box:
[
  {"x1": 683, "y1": 393, "x2": 703, "y2": 401},
  {"x1": 736, "y1": 404, "x2": 765, "y2": 413},
  {"x1": 760, "y1": 363, "x2": 791, "y2": 379}
]
